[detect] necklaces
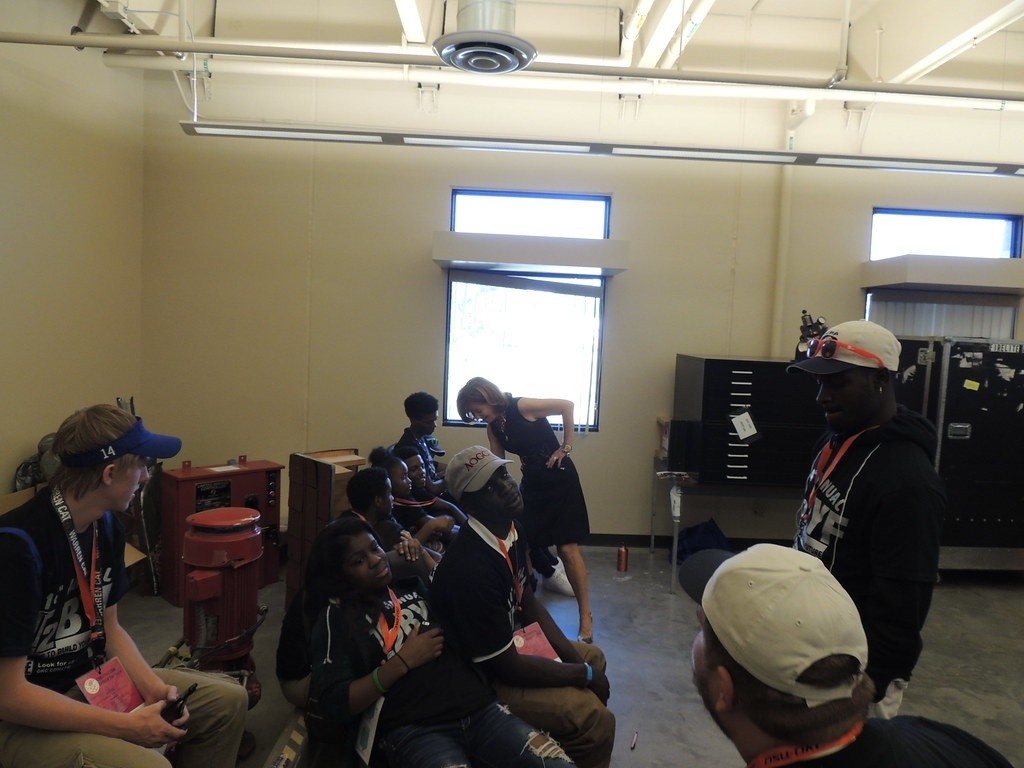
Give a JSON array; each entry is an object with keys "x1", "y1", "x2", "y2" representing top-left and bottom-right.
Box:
[{"x1": 498, "y1": 412, "x2": 508, "y2": 440}]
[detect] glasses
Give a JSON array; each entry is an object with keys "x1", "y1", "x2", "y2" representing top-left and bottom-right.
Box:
[{"x1": 807, "y1": 336, "x2": 887, "y2": 372}]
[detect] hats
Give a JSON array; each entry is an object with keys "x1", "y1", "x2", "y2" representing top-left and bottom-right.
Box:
[
  {"x1": 784, "y1": 318, "x2": 902, "y2": 385},
  {"x1": 675, "y1": 539, "x2": 869, "y2": 707},
  {"x1": 445, "y1": 444, "x2": 515, "y2": 500},
  {"x1": 64, "y1": 414, "x2": 183, "y2": 462}
]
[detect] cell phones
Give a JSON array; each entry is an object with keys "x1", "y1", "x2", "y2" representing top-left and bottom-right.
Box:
[
  {"x1": 161, "y1": 682, "x2": 198, "y2": 718},
  {"x1": 417, "y1": 619, "x2": 441, "y2": 636}
]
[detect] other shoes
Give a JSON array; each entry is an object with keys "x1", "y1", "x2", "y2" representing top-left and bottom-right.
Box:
[
  {"x1": 577, "y1": 614, "x2": 594, "y2": 644},
  {"x1": 554, "y1": 557, "x2": 568, "y2": 576},
  {"x1": 542, "y1": 570, "x2": 577, "y2": 597}
]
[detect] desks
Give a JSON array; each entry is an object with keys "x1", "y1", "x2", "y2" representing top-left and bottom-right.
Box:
[{"x1": 652, "y1": 456, "x2": 809, "y2": 593}]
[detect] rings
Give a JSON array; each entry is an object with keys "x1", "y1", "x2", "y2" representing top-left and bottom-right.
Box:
[{"x1": 554, "y1": 457, "x2": 557, "y2": 459}]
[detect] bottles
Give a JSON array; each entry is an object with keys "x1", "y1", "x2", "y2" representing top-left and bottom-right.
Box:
[{"x1": 617, "y1": 541, "x2": 629, "y2": 572}]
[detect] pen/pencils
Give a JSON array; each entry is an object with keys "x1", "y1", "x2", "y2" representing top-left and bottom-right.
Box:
[{"x1": 631, "y1": 732, "x2": 638, "y2": 749}]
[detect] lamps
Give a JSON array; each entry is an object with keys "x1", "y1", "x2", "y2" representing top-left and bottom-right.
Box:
[{"x1": 179, "y1": 48, "x2": 1024, "y2": 179}]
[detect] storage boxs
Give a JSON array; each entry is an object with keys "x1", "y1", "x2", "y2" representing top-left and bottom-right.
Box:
[{"x1": 655, "y1": 416, "x2": 671, "y2": 461}]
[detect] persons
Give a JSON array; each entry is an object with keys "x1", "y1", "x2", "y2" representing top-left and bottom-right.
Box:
[
  {"x1": 456, "y1": 377, "x2": 593, "y2": 643},
  {"x1": 0, "y1": 403, "x2": 249, "y2": 768},
  {"x1": 427, "y1": 445, "x2": 615, "y2": 768},
  {"x1": 787, "y1": 318, "x2": 967, "y2": 719},
  {"x1": 678, "y1": 543, "x2": 1015, "y2": 768},
  {"x1": 275, "y1": 391, "x2": 581, "y2": 768}
]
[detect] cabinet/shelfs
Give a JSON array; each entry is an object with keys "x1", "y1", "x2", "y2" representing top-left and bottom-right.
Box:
[
  {"x1": 896, "y1": 337, "x2": 1024, "y2": 582},
  {"x1": 669, "y1": 353, "x2": 830, "y2": 489}
]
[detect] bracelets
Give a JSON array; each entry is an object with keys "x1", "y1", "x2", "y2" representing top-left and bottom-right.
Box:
[
  {"x1": 395, "y1": 653, "x2": 411, "y2": 671},
  {"x1": 561, "y1": 443, "x2": 572, "y2": 453},
  {"x1": 585, "y1": 662, "x2": 592, "y2": 681},
  {"x1": 372, "y1": 667, "x2": 389, "y2": 693}
]
